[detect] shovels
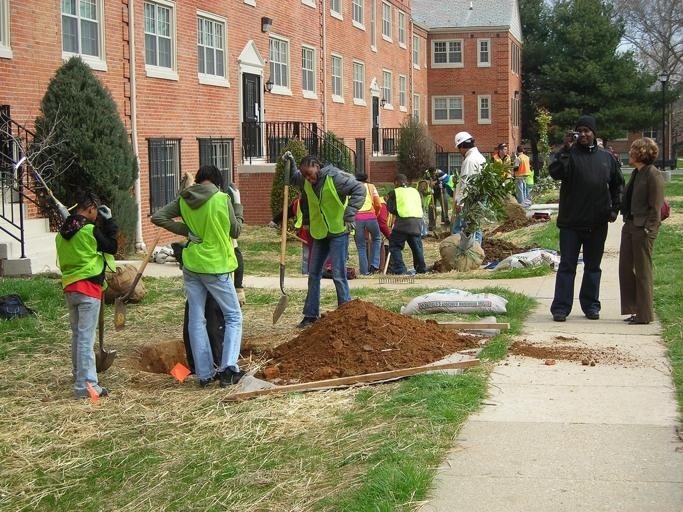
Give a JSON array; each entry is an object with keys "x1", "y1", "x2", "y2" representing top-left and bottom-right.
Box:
[
  {"x1": 440, "y1": 182, "x2": 451, "y2": 233},
  {"x1": 431, "y1": 196, "x2": 438, "y2": 239},
  {"x1": 273, "y1": 157, "x2": 290, "y2": 324},
  {"x1": 114, "y1": 227, "x2": 166, "y2": 332},
  {"x1": 96, "y1": 291, "x2": 116, "y2": 373}
]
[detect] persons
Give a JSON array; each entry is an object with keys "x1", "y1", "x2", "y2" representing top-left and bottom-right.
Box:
[
  {"x1": 150, "y1": 164, "x2": 245, "y2": 388},
  {"x1": 453, "y1": 133, "x2": 489, "y2": 244},
  {"x1": 493, "y1": 144, "x2": 535, "y2": 207},
  {"x1": 281, "y1": 150, "x2": 366, "y2": 327},
  {"x1": 607, "y1": 146, "x2": 621, "y2": 166},
  {"x1": 619, "y1": 135, "x2": 665, "y2": 324},
  {"x1": 549, "y1": 115, "x2": 626, "y2": 321},
  {"x1": 292, "y1": 192, "x2": 312, "y2": 275},
  {"x1": 349, "y1": 168, "x2": 457, "y2": 273},
  {"x1": 171, "y1": 236, "x2": 245, "y2": 375},
  {"x1": 56, "y1": 187, "x2": 119, "y2": 397}
]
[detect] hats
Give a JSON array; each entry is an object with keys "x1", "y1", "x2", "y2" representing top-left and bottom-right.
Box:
[{"x1": 575, "y1": 115, "x2": 597, "y2": 137}]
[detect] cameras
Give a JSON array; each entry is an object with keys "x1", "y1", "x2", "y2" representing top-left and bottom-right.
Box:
[{"x1": 570, "y1": 132, "x2": 580, "y2": 140}]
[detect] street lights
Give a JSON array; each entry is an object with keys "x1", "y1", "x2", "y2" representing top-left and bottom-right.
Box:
[{"x1": 657, "y1": 69, "x2": 670, "y2": 173}]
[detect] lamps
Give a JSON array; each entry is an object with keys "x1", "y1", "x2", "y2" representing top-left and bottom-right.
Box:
[
  {"x1": 379, "y1": 97, "x2": 387, "y2": 108},
  {"x1": 260, "y1": 16, "x2": 272, "y2": 32},
  {"x1": 263, "y1": 76, "x2": 274, "y2": 95}
]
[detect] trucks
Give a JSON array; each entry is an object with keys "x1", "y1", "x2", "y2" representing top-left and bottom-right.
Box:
[{"x1": 652, "y1": 159, "x2": 676, "y2": 171}]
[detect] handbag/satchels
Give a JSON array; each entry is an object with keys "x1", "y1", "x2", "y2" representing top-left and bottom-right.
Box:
[{"x1": 660, "y1": 202, "x2": 671, "y2": 222}]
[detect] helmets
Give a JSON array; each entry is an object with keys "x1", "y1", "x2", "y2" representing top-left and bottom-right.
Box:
[{"x1": 453, "y1": 131, "x2": 472, "y2": 148}]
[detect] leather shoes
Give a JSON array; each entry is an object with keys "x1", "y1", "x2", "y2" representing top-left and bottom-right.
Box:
[
  {"x1": 552, "y1": 315, "x2": 565, "y2": 322},
  {"x1": 623, "y1": 315, "x2": 649, "y2": 324},
  {"x1": 587, "y1": 314, "x2": 599, "y2": 320}
]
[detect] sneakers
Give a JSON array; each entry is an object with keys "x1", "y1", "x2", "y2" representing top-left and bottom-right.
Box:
[
  {"x1": 296, "y1": 315, "x2": 316, "y2": 327},
  {"x1": 219, "y1": 368, "x2": 246, "y2": 388},
  {"x1": 199, "y1": 370, "x2": 220, "y2": 387}
]
